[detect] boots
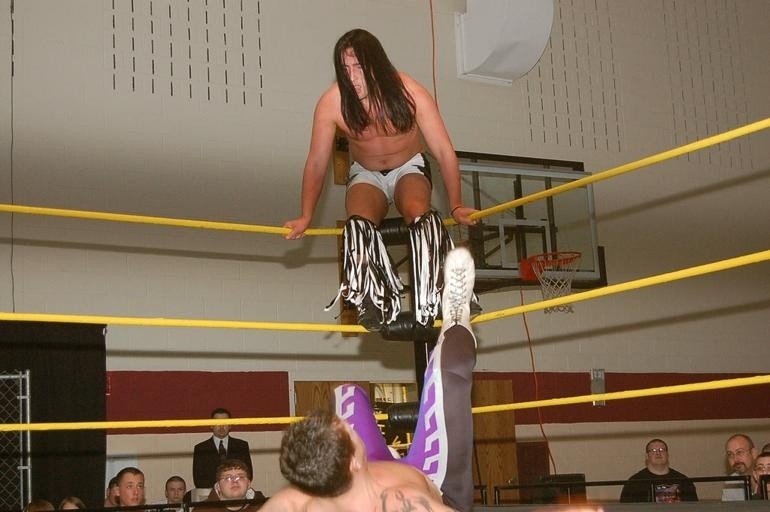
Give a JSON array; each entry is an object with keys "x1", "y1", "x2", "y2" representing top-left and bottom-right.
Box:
[
  {"x1": 353, "y1": 262, "x2": 390, "y2": 328},
  {"x1": 428, "y1": 265, "x2": 484, "y2": 324},
  {"x1": 432, "y1": 242, "x2": 485, "y2": 349}
]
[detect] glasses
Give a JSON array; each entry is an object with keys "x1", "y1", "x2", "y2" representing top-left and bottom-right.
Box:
[
  {"x1": 727, "y1": 447, "x2": 752, "y2": 459},
  {"x1": 648, "y1": 447, "x2": 667, "y2": 453},
  {"x1": 218, "y1": 474, "x2": 250, "y2": 483},
  {"x1": 753, "y1": 463, "x2": 770, "y2": 472}
]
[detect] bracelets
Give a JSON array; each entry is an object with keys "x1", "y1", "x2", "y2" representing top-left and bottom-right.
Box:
[{"x1": 450, "y1": 205, "x2": 462, "y2": 215}]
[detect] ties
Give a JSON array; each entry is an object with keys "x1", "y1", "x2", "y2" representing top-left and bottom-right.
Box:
[{"x1": 217, "y1": 439, "x2": 227, "y2": 459}]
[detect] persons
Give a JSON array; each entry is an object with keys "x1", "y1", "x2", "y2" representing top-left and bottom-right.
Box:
[
  {"x1": 258, "y1": 246, "x2": 478, "y2": 512},
  {"x1": 619, "y1": 438, "x2": 699, "y2": 502},
  {"x1": 721, "y1": 433, "x2": 770, "y2": 501},
  {"x1": 193, "y1": 408, "x2": 253, "y2": 488},
  {"x1": 282, "y1": 28, "x2": 482, "y2": 334},
  {"x1": 21, "y1": 459, "x2": 270, "y2": 512}
]
